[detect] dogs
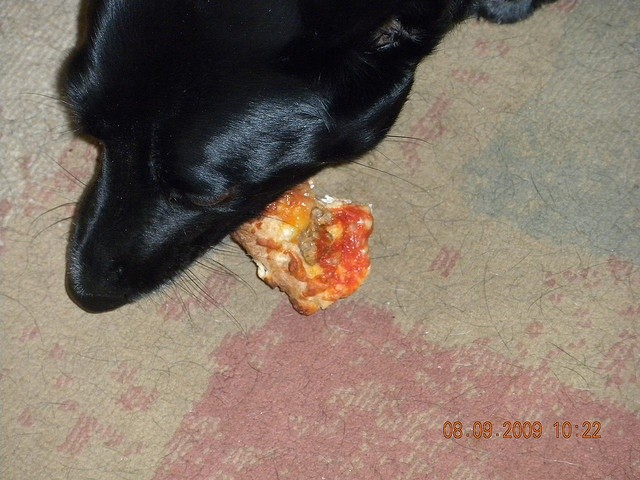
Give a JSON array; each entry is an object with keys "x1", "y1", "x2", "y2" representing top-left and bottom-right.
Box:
[{"x1": 19, "y1": 0, "x2": 563, "y2": 335}]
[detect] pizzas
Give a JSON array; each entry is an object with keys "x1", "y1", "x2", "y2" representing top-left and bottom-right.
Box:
[{"x1": 229, "y1": 177, "x2": 376, "y2": 316}]
